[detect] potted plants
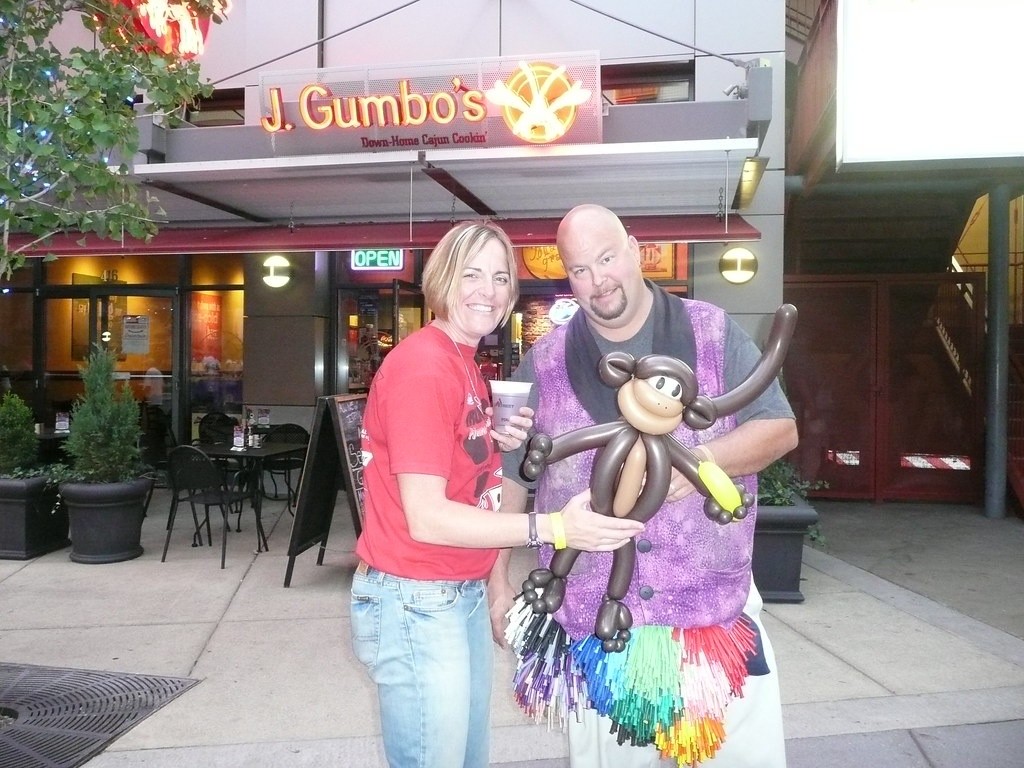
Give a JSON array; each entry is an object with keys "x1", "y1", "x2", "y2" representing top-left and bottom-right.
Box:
[
  {"x1": 42, "y1": 341, "x2": 154, "y2": 564},
  {"x1": 0, "y1": 388, "x2": 72, "y2": 560},
  {"x1": 751, "y1": 457, "x2": 831, "y2": 603}
]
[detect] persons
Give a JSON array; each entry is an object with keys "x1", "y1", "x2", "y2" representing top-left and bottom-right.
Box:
[
  {"x1": 142, "y1": 358, "x2": 163, "y2": 408},
  {"x1": 193, "y1": 352, "x2": 220, "y2": 377},
  {"x1": 350, "y1": 221, "x2": 646, "y2": 768},
  {"x1": 358, "y1": 335, "x2": 368, "y2": 346},
  {"x1": 485, "y1": 202, "x2": 801, "y2": 768}
]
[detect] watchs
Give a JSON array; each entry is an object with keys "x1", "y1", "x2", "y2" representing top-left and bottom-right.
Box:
[{"x1": 525, "y1": 512, "x2": 544, "y2": 551}]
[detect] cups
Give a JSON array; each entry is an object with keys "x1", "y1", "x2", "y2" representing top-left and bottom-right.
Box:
[{"x1": 488, "y1": 378, "x2": 534, "y2": 436}]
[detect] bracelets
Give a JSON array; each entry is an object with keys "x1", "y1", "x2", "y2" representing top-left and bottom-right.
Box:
[
  {"x1": 694, "y1": 444, "x2": 716, "y2": 464},
  {"x1": 550, "y1": 512, "x2": 566, "y2": 550}
]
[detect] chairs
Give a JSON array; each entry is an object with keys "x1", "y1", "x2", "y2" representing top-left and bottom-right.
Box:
[
  {"x1": 190, "y1": 411, "x2": 240, "y2": 514},
  {"x1": 145, "y1": 406, "x2": 179, "y2": 489},
  {"x1": 245, "y1": 423, "x2": 310, "y2": 508},
  {"x1": 161, "y1": 444, "x2": 261, "y2": 569}
]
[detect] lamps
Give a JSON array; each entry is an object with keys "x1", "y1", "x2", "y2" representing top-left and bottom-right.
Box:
[
  {"x1": 261, "y1": 255, "x2": 295, "y2": 288},
  {"x1": 719, "y1": 247, "x2": 758, "y2": 285}
]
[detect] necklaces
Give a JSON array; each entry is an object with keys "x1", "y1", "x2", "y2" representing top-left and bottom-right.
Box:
[{"x1": 437, "y1": 321, "x2": 484, "y2": 415}]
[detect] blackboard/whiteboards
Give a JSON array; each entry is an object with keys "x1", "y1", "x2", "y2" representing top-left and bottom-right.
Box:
[{"x1": 327, "y1": 392, "x2": 368, "y2": 539}]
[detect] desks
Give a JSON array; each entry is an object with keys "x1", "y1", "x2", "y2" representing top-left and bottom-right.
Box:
[
  {"x1": 192, "y1": 442, "x2": 309, "y2": 552},
  {"x1": 234, "y1": 423, "x2": 296, "y2": 513},
  {"x1": 32, "y1": 427, "x2": 70, "y2": 459}
]
